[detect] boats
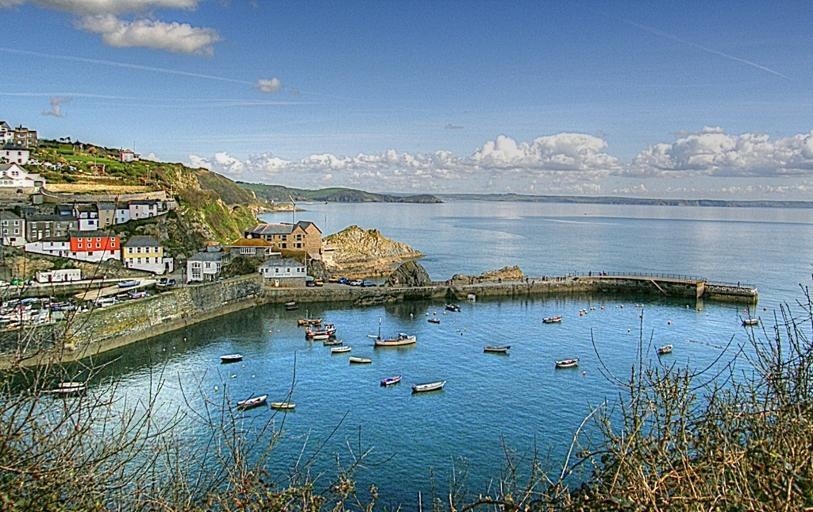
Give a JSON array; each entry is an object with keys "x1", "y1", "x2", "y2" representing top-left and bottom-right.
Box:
[
  {"x1": 268, "y1": 400, "x2": 296, "y2": 410},
  {"x1": 235, "y1": 390, "x2": 269, "y2": 410},
  {"x1": 483, "y1": 343, "x2": 510, "y2": 353},
  {"x1": 219, "y1": 353, "x2": 243, "y2": 362},
  {"x1": 658, "y1": 345, "x2": 671, "y2": 354},
  {"x1": 554, "y1": 356, "x2": 581, "y2": 370},
  {"x1": 282, "y1": 300, "x2": 462, "y2": 395},
  {"x1": 36, "y1": 379, "x2": 87, "y2": 398},
  {"x1": 741, "y1": 318, "x2": 759, "y2": 326},
  {"x1": 541, "y1": 313, "x2": 563, "y2": 323}
]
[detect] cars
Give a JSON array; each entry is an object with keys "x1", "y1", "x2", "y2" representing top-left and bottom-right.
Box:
[
  {"x1": 0, "y1": 264, "x2": 177, "y2": 331},
  {"x1": 304, "y1": 273, "x2": 378, "y2": 288}
]
